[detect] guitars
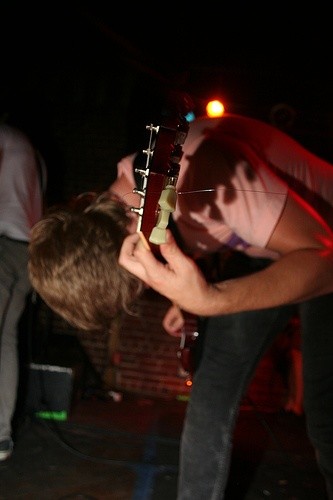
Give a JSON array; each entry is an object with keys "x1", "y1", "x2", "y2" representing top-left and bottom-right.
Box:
[{"x1": 106, "y1": 110, "x2": 207, "y2": 397}]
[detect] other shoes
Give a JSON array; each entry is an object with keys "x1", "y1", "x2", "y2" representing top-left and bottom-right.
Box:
[{"x1": 0, "y1": 440, "x2": 13, "y2": 461}]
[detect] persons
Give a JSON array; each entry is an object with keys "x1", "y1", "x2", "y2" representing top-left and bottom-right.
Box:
[
  {"x1": 25, "y1": 110, "x2": 333, "y2": 500},
  {"x1": 0, "y1": 126, "x2": 46, "y2": 464}
]
